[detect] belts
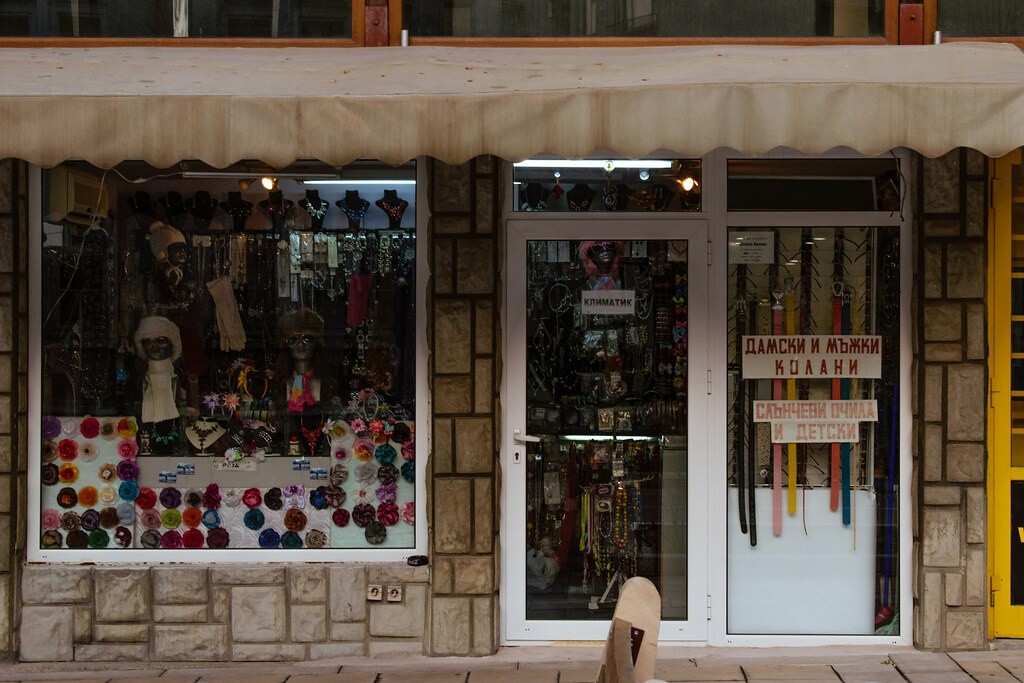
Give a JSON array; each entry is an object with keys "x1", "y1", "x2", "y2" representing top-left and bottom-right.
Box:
[
  {"x1": 841, "y1": 291, "x2": 851, "y2": 526},
  {"x1": 829, "y1": 281, "x2": 843, "y2": 514},
  {"x1": 748, "y1": 294, "x2": 757, "y2": 549},
  {"x1": 772, "y1": 286, "x2": 785, "y2": 538},
  {"x1": 734, "y1": 300, "x2": 748, "y2": 536},
  {"x1": 788, "y1": 283, "x2": 799, "y2": 515}
]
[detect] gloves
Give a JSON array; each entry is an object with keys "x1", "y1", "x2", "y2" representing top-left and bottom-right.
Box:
[{"x1": 206, "y1": 275, "x2": 247, "y2": 351}]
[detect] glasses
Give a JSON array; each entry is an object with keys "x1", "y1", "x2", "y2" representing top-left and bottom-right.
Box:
[
  {"x1": 590, "y1": 241, "x2": 618, "y2": 254},
  {"x1": 285, "y1": 334, "x2": 317, "y2": 347},
  {"x1": 140, "y1": 335, "x2": 169, "y2": 346},
  {"x1": 169, "y1": 244, "x2": 187, "y2": 254}
]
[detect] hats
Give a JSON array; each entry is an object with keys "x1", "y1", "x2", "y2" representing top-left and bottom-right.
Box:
[
  {"x1": 133, "y1": 308, "x2": 185, "y2": 362},
  {"x1": 149, "y1": 221, "x2": 188, "y2": 286},
  {"x1": 273, "y1": 308, "x2": 331, "y2": 368}
]
